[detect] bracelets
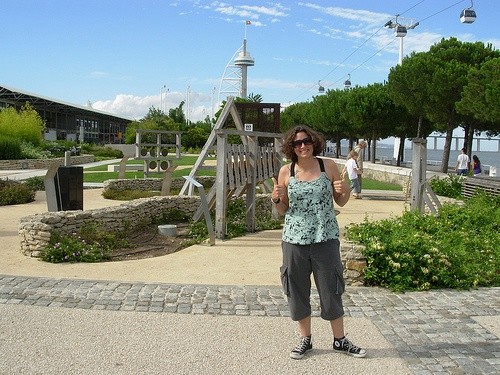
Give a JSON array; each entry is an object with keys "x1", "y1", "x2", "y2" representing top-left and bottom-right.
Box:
[{"x1": 270, "y1": 198, "x2": 281, "y2": 205}]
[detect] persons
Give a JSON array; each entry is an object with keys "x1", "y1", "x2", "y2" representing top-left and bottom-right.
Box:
[
  {"x1": 343, "y1": 141, "x2": 367, "y2": 199},
  {"x1": 340, "y1": 149, "x2": 360, "y2": 199},
  {"x1": 472, "y1": 155, "x2": 482, "y2": 174},
  {"x1": 272, "y1": 125, "x2": 367, "y2": 360},
  {"x1": 455, "y1": 148, "x2": 470, "y2": 177}
]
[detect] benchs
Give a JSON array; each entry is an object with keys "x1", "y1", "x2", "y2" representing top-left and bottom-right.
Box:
[{"x1": 108, "y1": 164, "x2": 144, "y2": 172}]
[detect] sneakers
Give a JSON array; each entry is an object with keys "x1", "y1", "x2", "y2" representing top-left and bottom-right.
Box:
[
  {"x1": 332, "y1": 332, "x2": 367, "y2": 357},
  {"x1": 289, "y1": 334, "x2": 313, "y2": 359}
]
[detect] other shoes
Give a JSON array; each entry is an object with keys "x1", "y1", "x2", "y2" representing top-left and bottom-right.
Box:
[{"x1": 352, "y1": 193, "x2": 362, "y2": 198}]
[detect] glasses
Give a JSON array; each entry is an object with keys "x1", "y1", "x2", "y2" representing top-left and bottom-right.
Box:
[{"x1": 291, "y1": 138, "x2": 313, "y2": 148}]
